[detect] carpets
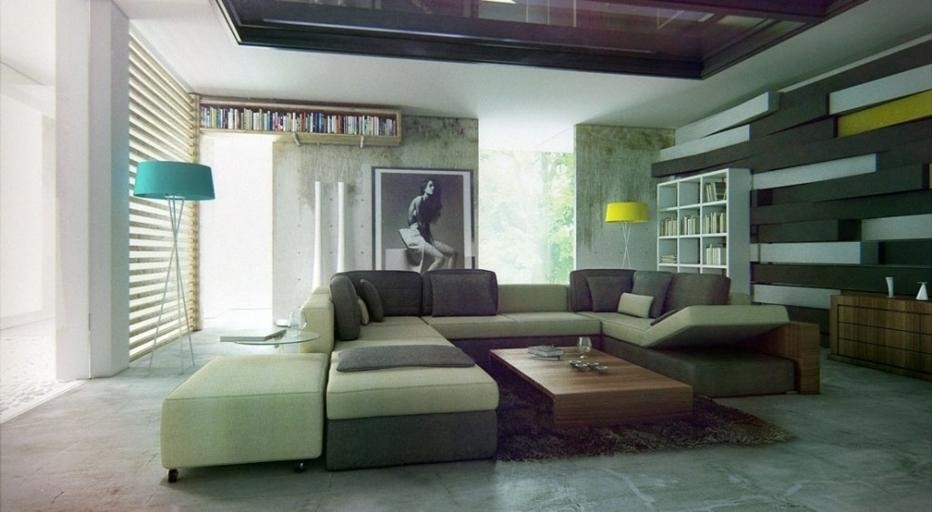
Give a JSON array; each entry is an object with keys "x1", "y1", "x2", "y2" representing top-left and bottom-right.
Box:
[{"x1": 478, "y1": 363, "x2": 796, "y2": 457}]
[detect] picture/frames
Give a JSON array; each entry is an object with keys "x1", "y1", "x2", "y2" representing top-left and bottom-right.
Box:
[{"x1": 372, "y1": 166, "x2": 474, "y2": 271}]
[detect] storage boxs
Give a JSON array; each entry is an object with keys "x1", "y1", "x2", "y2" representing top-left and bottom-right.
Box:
[{"x1": 827, "y1": 292, "x2": 932, "y2": 382}]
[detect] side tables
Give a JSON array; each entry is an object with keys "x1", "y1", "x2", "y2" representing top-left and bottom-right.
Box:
[{"x1": 232, "y1": 327, "x2": 321, "y2": 352}]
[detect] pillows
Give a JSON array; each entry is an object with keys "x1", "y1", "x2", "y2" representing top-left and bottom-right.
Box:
[
  {"x1": 360, "y1": 279, "x2": 383, "y2": 322},
  {"x1": 357, "y1": 297, "x2": 369, "y2": 325},
  {"x1": 585, "y1": 276, "x2": 632, "y2": 313},
  {"x1": 617, "y1": 291, "x2": 654, "y2": 320},
  {"x1": 633, "y1": 271, "x2": 675, "y2": 320},
  {"x1": 336, "y1": 344, "x2": 476, "y2": 374}
]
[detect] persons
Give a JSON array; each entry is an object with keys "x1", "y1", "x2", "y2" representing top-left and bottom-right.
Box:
[{"x1": 407, "y1": 179, "x2": 458, "y2": 271}]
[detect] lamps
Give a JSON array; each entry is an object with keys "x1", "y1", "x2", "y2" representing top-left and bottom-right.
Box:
[
  {"x1": 605, "y1": 201, "x2": 650, "y2": 269},
  {"x1": 133, "y1": 160, "x2": 215, "y2": 370}
]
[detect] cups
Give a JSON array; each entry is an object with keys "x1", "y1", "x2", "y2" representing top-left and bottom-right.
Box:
[
  {"x1": 569, "y1": 360, "x2": 581, "y2": 368},
  {"x1": 576, "y1": 363, "x2": 587, "y2": 371},
  {"x1": 587, "y1": 362, "x2": 598, "y2": 370},
  {"x1": 576, "y1": 337, "x2": 592, "y2": 359},
  {"x1": 597, "y1": 365, "x2": 608, "y2": 373}
]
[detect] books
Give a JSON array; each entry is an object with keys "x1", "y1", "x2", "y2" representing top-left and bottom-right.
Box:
[
  {"x1": 703, "y1": 212, "x2": 726, "y2": 233},
  {"x1": 679, "y1": 215, "x2": 699, "y2": 234},
  {"x1": 704, "y1": 244, "x2": 726, "y2": 266},
  {"x1": 661, "y1": 253, "x2": 676, "y2": 265},
  {"x1": 200, "y1": 107, "x2": 395, "y2": 136},
  {"x1": 660, "y1": 217, "x2": 677, "y2": 235},
  {"x1": 529, "y1": 344, "x2": 565, "y2": 361},
  {"x1": 705, "y1": 182, "x2": 725, "y2": 201},
  {"x1": 220, "y1": 327, "x2": 286, "y2": 341}
]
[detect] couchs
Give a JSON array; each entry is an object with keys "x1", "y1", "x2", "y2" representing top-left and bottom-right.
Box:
[{"x1": 159, "y1": 268, "x2": 822, "y2": 484}]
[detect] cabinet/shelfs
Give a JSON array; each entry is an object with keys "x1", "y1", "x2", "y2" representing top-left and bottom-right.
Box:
[
  {"x1": 199, "y1": 98, "x2": 401, "y2": 149},
  {"x1": 656, "y1": 168, "x2": 752, "y2": 295}
]
